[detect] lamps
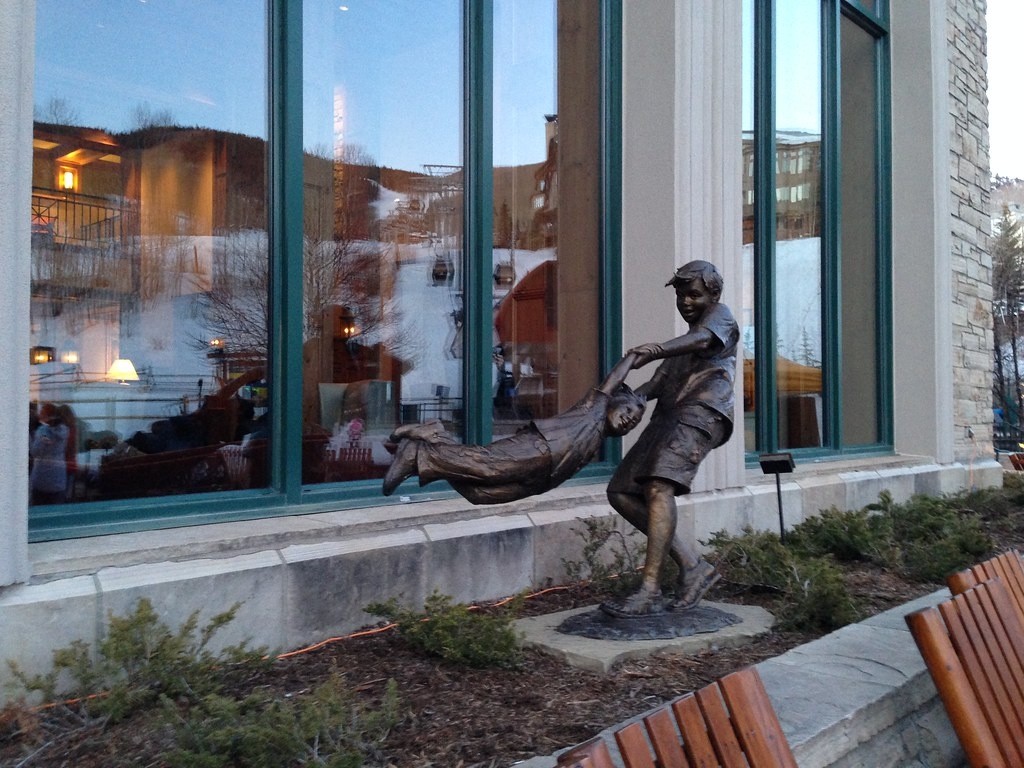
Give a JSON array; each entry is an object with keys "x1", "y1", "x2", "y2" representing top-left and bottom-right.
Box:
[{"x1": 104, "y1": 359, "x2": 140, "y2": 384}]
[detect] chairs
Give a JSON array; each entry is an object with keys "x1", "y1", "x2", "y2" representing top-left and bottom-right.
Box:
[
  {"x1": 554, "y1": 666, "x2": 799, "y2": 768},
  {"x1": 946, "y1": 550, "x2": 1024, "y2": 596},
  {"x1": 1008, "y1": 452, "x2": 1024, "y2": 471},
  {"x1": 905, "y1": 577, "x2": 1024, "y2": 768}
]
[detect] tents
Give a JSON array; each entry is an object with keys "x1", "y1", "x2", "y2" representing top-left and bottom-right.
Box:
[{"x1": 742, "y1": 353, "x2": 821, "y2": 410}]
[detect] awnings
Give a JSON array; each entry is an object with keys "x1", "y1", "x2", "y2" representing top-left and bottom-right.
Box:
[{"x1": 450, "y1": 261, "x2": 557, "y2": 368}]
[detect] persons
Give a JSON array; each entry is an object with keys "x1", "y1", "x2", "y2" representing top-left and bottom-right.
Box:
[
  {"x1": 350, "y1": 418, "x2": 361, "y2": 447},
  {"x1": 29, "y1": 403, "x2": 77, "y2": 506},
  {"x1": 384, "y1": 259, "x2": 741, "y2": 617},
  {"x1": 449, "y1": 302, "x2": 506, "y2": 419},
  {"x1": 993, "y1": 404, "x2": 1004, "y2": 419},
  {"x1": 121, "y1": 304, "x2": 386, "y2": 490}
]
